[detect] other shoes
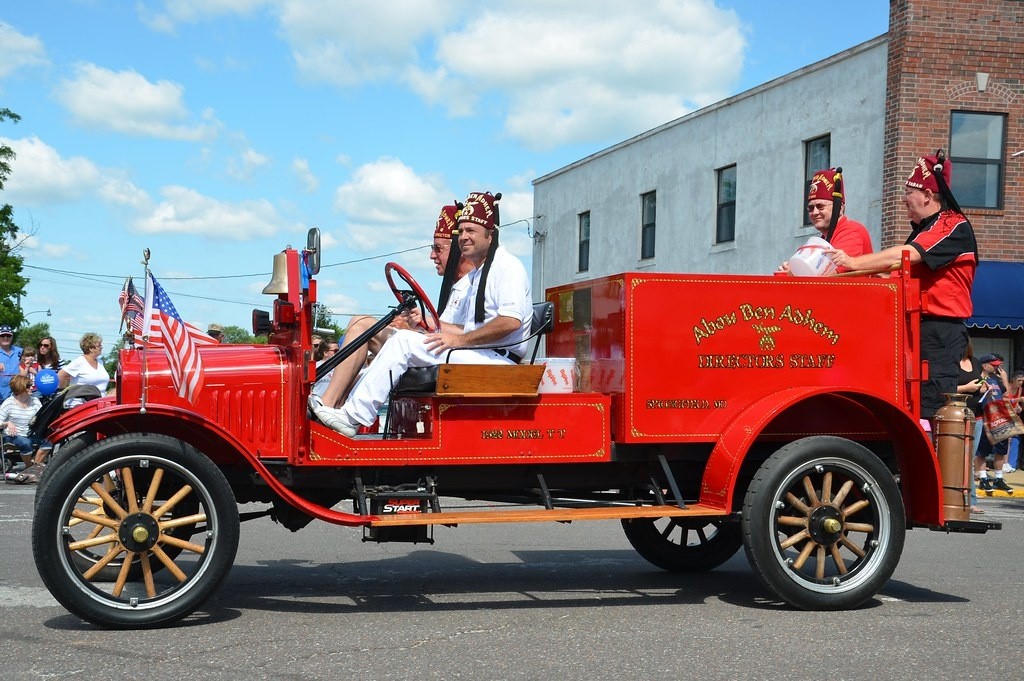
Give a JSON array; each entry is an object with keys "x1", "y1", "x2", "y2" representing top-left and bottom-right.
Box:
[
  {"x1": 970, "y1": 507, "x2": 984, "y2": 514},
  {"x1": 1003, "y1": 463, "x2": 1016, "y2": 473},
  {"x1": 975, "y1": 472, "x2": 994, "y2": 482}
]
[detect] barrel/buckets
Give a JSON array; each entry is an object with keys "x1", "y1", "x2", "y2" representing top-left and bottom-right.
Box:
[
  {"x1": 789, "y1": 236, "x2": 838, "y2": 277},
  {"x1": 589, "y1": 358, "x2": 625, "y2": 394},
  {"x1": 533, "y1": 357, "x2": 576, "y2": 394}
]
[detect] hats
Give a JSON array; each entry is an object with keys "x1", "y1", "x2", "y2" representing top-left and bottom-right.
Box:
[
  {"x1": 808, "y1": 167, "x2": 845, "y2": 243},
  {"x1": 0, "y1": 325, "x2": 13, "y2": 336},
  {"x1": 979, "y1": 354, "x2": 1001, "y2": 366},
  {"x1": 206, "y1": 323, "x2": 225, "y2": 335},
  {"x1": 457, "y1": 191, "x2": 502, "y2": 324},
  {"x1": 433, "y1": 200, "x2": 465, "y2": 318},
  {"x1": 905, "y1": 149, "x2": 979, "y2": 267}
]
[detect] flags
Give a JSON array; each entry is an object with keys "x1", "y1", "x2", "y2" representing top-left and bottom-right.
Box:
[
  {"x1": 142, "y1": 272, "x2": 205, "y2": 407},
  {"x1": 118, "y1": 276, "x2": 145, "y2": 333},
  {"x1": 134, "y1": 322, "x2": 218, "y2": 348}
]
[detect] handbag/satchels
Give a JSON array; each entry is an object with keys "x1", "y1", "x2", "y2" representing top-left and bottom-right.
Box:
[{"x1": 982, "y1": 395, "x2": 1024, "y2": 446}]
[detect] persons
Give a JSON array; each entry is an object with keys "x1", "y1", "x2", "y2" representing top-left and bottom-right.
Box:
[
  {"x1": 821, "y1": 149, "x2": 979, "y2": 441},
  {"x1": 207, "y1": 323, "x2": 225, "y2": 343},
  {"x1": 37, "y1": 336, "x2": 66, "y2": 395},
  {"x1": 774, "y1": 166, "x2": 879, "y2": 276},
  {"x1": 310, "y1": 339, "x2": 339, "y2": 398},
  {"x1": 0, "y1": 325, "x2": 24, "y2": 405},
  {"x1": 308, "y1": 191, "x2": 533, "y2": 438},
  {"x1": 58, "y1": 331, "x2": 110, "y2": 408},
  {"x1": 957, "y1": 337, "x2": 986, "y2": 514},
  {"x1": 311, "y1": 335, "x2": 324, "y2": 361},
  {"x1": 1003, "y1": 371, "x2": 1024, "y2": 473},
  {"x1": 0, "y1": 375, "x2": 53, "y2": 469},
  {"x1": 974, "y1": 353, "x2": 1014, "y2": 493},
  {"x1": 19, "y1": 346, "x2": 44, "y2": 405}
]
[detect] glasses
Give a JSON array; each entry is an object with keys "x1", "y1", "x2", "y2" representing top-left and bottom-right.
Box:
[
  {"x1": 312, "y1": 344, "x2": 319, "y2": 348},
  {"x1": 40, "y1": 344, "x2": 49, "y2": 347},
  {"x1": 323, "y1": 349, "x2": 338, "y2": 354},
  {"x1": 807, "y1": 203, "x2": 842, "y2": 212},
  {"x1": 431, "y1": 245, "x2": 451, "y2": 253},
  {"x1": 26, "y1": 384, "x2": 32, "y2": 389}
]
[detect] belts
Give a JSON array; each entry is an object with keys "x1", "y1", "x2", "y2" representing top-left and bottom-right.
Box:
[{"x1": 492, "y1": 348, "x2": 521, "y2": 364}]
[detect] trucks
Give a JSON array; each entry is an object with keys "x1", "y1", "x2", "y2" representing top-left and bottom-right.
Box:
[{"x1": 30, "y1": 224, "x2": 1004, "y2": 630}]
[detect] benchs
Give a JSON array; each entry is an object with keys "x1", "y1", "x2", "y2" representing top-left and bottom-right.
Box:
[{"x1": 394, "y1": 301, "x2": 555, "y2": 399}]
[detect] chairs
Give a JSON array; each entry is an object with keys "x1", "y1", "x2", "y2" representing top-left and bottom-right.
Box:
[{"x1": 0, "y1": 424, "x2": 52, "y2": 484}]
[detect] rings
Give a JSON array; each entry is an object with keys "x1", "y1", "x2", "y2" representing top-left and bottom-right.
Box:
[{"x1": 441, "y1": 340, "x2": 444, "y2": 345}]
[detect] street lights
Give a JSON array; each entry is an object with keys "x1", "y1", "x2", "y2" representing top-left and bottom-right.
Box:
[{"x1": 16, "y1": 293, "x2": 53, "y2": 347}]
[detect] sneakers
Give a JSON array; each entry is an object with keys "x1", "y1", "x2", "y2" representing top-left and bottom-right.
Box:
[
  {"x1": 992, "y1": 478, "x2": 1014, "y2": 492},
  {"x1": 979, "y1": 477, "x2": 994, "y2": 492},
  {"x1": 314, "y1": 405, "x2": 362, "y2": 439},
  {"x1": 307, "y1": 395, "x2": 324, "y2": 413}
]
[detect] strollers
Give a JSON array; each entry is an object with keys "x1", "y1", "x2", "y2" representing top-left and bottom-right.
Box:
[{"x1": 26, "y1": 384, "x2": 105, "y2": 486}]
